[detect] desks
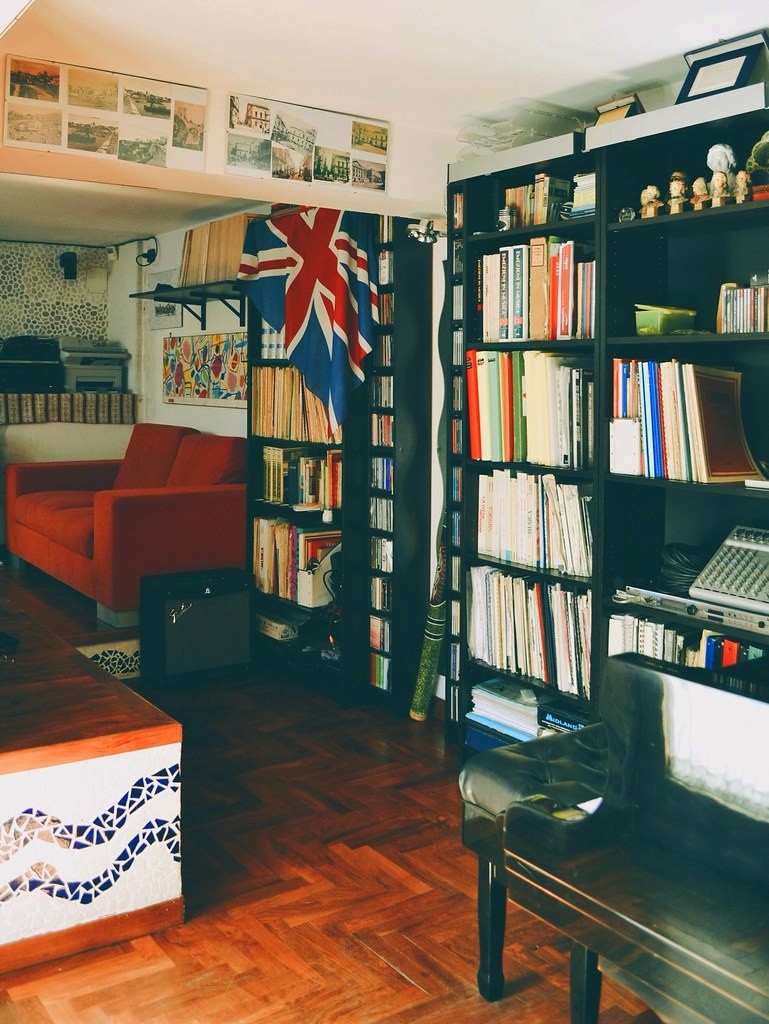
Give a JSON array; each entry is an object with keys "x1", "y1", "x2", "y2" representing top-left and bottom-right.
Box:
[{"x1": 1, "y1": 593, "x2": 185, "y2": 974}]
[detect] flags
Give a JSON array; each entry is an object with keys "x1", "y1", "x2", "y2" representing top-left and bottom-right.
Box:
[{"x1": 237, "y1": 208, "x2": 379, "y2": 440}]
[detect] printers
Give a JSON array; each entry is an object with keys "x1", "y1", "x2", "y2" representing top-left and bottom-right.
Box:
[{"x1": 52, "y1": 336, "x2": 129, "y2": 394}]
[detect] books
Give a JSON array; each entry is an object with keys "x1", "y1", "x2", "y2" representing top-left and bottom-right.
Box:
[
  {"x1": 717, "y1": 283, "x2": 769, "y2": 333},
  {"x1": 608, "y1": 358, "x2": 769, "y2": 490},
  {"x1": 449, "y1": 170, "x2": 594, "y2": 750},
  {"x1": 608, "y1": 612, "x2": 769, "y2": 704},
  {"x1": 250, "y1": 216, "x2": 395, "y2": 692}
]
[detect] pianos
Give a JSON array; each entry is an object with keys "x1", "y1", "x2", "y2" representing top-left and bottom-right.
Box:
[{"x1": 497, "y1": 651, "x2": 769, "y2": 1024}]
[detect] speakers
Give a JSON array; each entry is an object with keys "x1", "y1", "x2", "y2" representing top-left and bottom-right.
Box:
[{"x1": 138, "y1": 565, "x2": 256, "y2": 691}]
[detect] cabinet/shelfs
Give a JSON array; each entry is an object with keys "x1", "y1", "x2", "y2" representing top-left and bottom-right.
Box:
[
  {"x1": 241, "y1": 202, "x2": 424, "y2": 711},
  {"x1": 443, "y1": 100, "x2": 769, "y2": 764}
]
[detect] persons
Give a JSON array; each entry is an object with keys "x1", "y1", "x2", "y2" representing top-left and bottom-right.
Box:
[{"x1": 639, "y1": 144, "x2": 751, "y2": 218}]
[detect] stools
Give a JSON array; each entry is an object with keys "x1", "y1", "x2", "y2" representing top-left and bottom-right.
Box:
[{"x1": 459, "y1": 721, "x2": 605, "y2": 1024}]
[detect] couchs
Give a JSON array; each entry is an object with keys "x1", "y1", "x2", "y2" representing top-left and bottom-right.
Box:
[{"x1": 5, "y1": 421, "x2": 250, "y2": 630}]
